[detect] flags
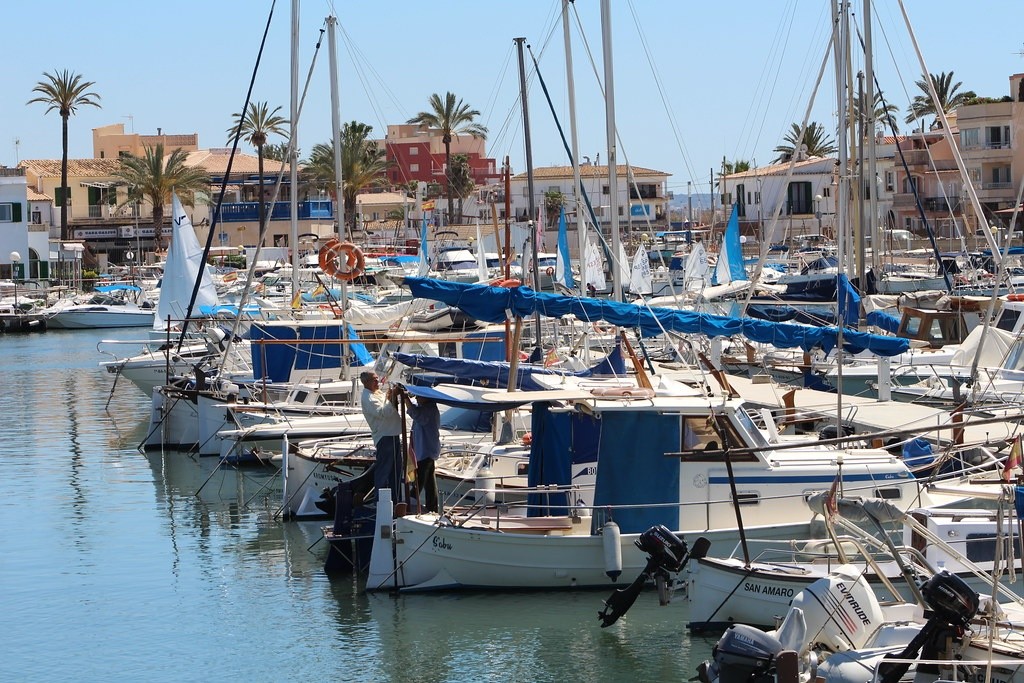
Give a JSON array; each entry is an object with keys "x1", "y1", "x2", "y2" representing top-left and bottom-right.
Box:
[
  {"x1": 1002, "y1": 435, "x2": 1022, "y2": 483},
  {"x1": 422, "y1": 200, "x2": 434, "y2": 211},
  {"x1": 544, "y1": 348, "x2": 559, "y2": 369},
  {"x1": 311, "y1": 284, "x2": 324, "y2": 298},
  {"x1": 223, "y1": 272, "x2": 237, "y2": 282},
  {"x1": 290, "y1": 289, "x2": 301, "y2": 309},
  {"x1": 256, "y1": 283, "x2": 264, "y2": 292}
]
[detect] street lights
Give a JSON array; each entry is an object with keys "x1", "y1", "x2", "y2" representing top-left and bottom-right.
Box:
[
  {"x1": 124, "y1": 251, "x2": 135, "y2": 287},
  {"x1": 10, "y1": 251, "x2": 21, "y2": 317}
]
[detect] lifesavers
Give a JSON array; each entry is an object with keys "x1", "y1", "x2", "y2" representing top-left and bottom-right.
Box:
[
  {"x1": 318, "y1": 238, "x2": 365, "y2": 280},
  {"x1": 591, "y1": 387, "x2": 656, "y2": 401},
  {"x1": 1007, "y1": 294, "x2": 1024, "y2": 300},
  {"x1": 952, "y1": 274, "x2": 968, "y2": 290},
  {"x1": 706, "y1": 255, "x2": 716, "y2": 264},
  {"x1": 490, "y1": 278, "x2": 521, "y2": 288}
]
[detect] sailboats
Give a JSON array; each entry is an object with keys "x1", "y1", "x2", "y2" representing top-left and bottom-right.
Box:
[{"x1": 0, "y1": 0, "x2": 1022, "y2": 683}]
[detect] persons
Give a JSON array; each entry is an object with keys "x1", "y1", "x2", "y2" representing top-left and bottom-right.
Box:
[
  {"x1": 360, "y1": 369, "x2": 402, "y2": 506},
  {"x1": 406, "y1": 395, "x2": 441, "y2": 512}
]
